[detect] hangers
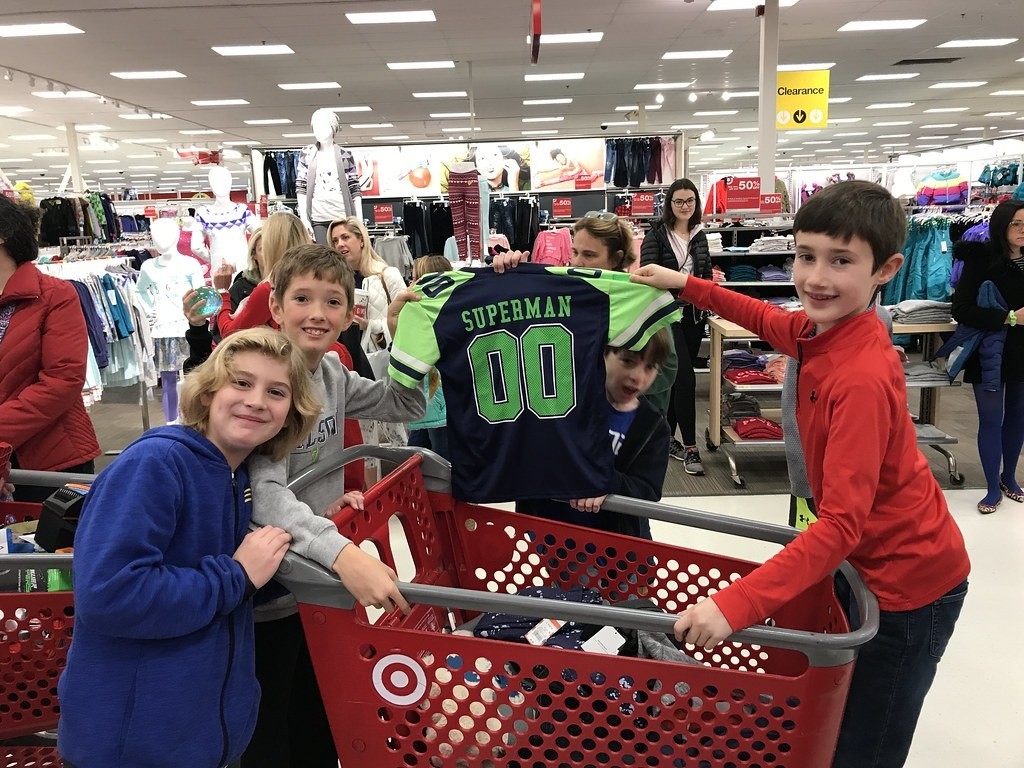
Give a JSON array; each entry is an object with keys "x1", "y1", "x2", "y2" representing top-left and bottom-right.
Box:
[
  {"x1": 614, "y1": 188, "x2": 635, "y2": 197},
  {"x1": 654, "y1": 188, "x2": 667, "y2": 198},
  {"x1": 382, "y1": 231, "x2": 396, "y2": 242},
  {"x1": 493, "y1": 192, "x2": 536, "y2": 203},
  {"x1": 909, "y1": 204, "x2": 997, "y2": 227},
  {"x1": 405, "y1": 195, "x2": 450, "y2": 206},
  {"x1": 35, "y1": 255, "x2": 140, "y2": 281}
]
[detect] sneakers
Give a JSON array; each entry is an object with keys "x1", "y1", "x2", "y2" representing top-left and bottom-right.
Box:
[
  {"x1": 683, "y1": 448, "x2": 705, "y2": 476},
  {"x1": 669, "y1": 437, "x2": 685, "y2": 461}
]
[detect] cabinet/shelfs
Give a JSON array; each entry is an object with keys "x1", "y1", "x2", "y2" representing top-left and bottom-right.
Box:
[
  {"x1": 693, "y1": 225, "x2": 802, "y2": 373},
  {"x1": 706, "y1": 316, "x2": 966, "y2": 490}
]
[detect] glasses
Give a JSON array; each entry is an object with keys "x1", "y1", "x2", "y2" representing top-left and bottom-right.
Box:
[
  {"x1": 671, "y1": 196, "x2": 697, "y2": 208},
  {"x1": 584, "y1": 211, "x2": 624, "y2": 249},
  {"x1": 1007, "y1": 221, "x2": 1024, "y2": 230}
]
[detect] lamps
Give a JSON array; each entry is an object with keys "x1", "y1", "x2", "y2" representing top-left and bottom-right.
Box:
[{"x1": 4, "y1": 68, "x2": 163, "y2": 158}]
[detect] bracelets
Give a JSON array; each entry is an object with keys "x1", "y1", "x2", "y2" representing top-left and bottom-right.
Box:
[{"x1": 1009, "y1": 310, "x2": 1017, "y2": 328}]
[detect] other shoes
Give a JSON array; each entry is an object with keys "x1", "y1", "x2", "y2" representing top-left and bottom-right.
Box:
[
  {"x1": 1000, "y1": 473, "x2": 1024, "y2": 502},
  {"x1": 977, "y1": 491, "x2": 1002, "y2": 514}
]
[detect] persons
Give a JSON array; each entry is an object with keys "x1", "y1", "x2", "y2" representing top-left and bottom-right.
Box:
[
  {"x1": 189, "y1": 165, "x2": 261, "y2": 285},
  {"x1": 134, "y1": 216, "x2": 205, "y2": 424},
  {"x1": 182, "y1": 182, "x2": 727, "y2": 768},
  {"x1": 445, "y1": 162, "x2": 482, "y2": 268},
  {"x1": 460, "y1": 142, "x2": 530, "y2": 190},
  {"x1": 630, "y1": 181, "x2": 972, "y2": 768},
  {"x1": 295, "y1": 110, "x2": 365, "y2": 242},
  {"x1": 950, "y1": 201, "x2": 1024, "y2": 515},
  {"x1": 530, "y1": 151, "x2": 604, "y2": 189},
  {"x1": 57, "y1": 323, "x2": 325, "y2": 767},
  {"x1": 1, "y1": 191, "x2": 102, "y2": 502}
]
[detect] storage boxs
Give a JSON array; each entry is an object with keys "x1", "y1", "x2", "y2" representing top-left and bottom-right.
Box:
[{"x1": 47, "y1": 569, "x2": 75, "y2": 591}]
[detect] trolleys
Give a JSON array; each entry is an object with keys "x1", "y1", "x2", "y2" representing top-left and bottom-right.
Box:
[
  {"x1": 0, "y1": 465, "x2": 98, "y2": 768},
  {"x1": 247, "y1": 443, "x2": 880, "y2": 768}
]
